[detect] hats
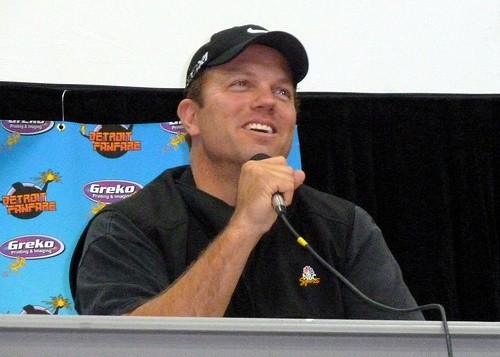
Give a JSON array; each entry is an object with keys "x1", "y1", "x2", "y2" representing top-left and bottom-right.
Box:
[{"x1": 185, "y1": 22, "x2": 310, "y2": 87}]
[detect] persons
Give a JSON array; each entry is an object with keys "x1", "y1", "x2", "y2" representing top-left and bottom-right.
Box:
[{"x1": 67, "y1": 25, "x2": 426, "y2": 322}]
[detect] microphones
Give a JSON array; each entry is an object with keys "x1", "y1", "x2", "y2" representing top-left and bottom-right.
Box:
[{"x1": 251, "y1": 153, "x2": 287, "y2": 217}]
